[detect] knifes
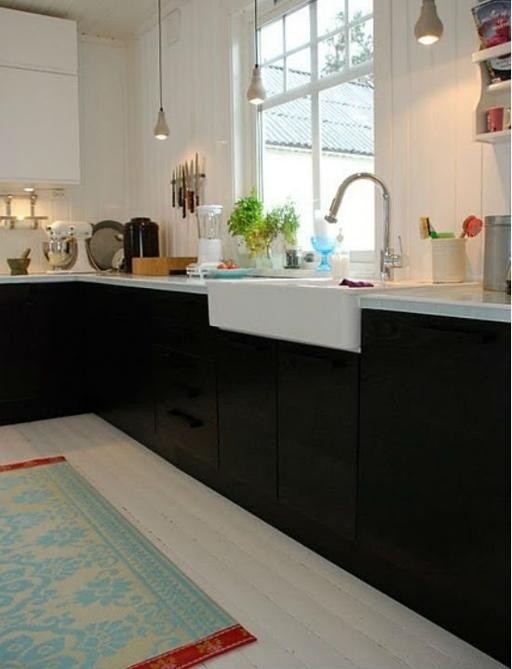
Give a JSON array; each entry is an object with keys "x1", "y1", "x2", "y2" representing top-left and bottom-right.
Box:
[{"x1": 171, "y1": 153, "x2": 201, "y2": 218}]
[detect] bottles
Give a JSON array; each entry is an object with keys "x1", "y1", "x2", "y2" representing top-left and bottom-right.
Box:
[{"x1": 124, "y1": 216, "x2": 159, "y2": 272}]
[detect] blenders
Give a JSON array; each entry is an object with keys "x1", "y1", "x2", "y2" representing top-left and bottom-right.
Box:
[{"x1": 186, "y1": 205, "x2": 225, "y2": 277}]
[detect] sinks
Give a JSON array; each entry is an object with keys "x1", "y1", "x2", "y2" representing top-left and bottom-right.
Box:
[{"x1": 208, "y1": 277, "x2": 429, "y2": 353}]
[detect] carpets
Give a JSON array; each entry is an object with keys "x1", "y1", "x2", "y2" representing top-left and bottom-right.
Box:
[{"x1": 1, "y1": 452, "x2": 256, "y2": 667}]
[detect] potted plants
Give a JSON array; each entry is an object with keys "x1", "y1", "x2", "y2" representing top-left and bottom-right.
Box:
[{"x1": 228, "y1": 184, "x2": 303, "y2": 272}]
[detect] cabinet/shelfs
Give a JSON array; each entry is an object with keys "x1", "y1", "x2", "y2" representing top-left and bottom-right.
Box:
[
  {"x1": 355, "y1": 316, "x2": 508, "y2": 599},
  {"x1": 93, "y1": 282, "x2": 141, "y2": 442},
  {"x1": 1, "y1": 282, "x2": 94, "y2": 428},
  {"x1": 1, "y1": 7, "x2": 82, "y2": 190},
  {"x1": 215, "y1": 336, "x2": 359, "y2": 546},
  {"x1": 142, "y1": 290, "x2": 220, "y2": 467},
  {"x1": 473, "y1": 0, "x2": 511, "y2": 144}
]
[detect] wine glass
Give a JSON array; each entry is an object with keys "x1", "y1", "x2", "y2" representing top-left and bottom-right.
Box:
[{"x1": 310, "y1": 237, "x2": 336, "y2": 270}]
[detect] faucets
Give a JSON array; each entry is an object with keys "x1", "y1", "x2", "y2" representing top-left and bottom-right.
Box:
[{"x1": 324, "y1": 172, "x2": 406, "y2": 280}]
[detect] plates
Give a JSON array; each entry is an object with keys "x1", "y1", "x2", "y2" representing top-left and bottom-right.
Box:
[{"x1": 210, "y1": 268, "x2": 254, "y2": 277}]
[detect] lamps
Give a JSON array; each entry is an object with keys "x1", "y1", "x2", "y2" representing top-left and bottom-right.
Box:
[
  {"x1": 152, "y1": 1, "x2": 169, "y2": 141},
  {"x1": 247, "y1": 0, "x2": 268, "y2": 107},
  {"x1": 411, "y1": 0, "x2": 445, "y2": 48}
]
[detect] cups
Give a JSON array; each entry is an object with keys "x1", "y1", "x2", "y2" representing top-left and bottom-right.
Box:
[
  {"x1": 432, "y1": 238, "x2": 466, "y2": 284},
  {"x1": 484, "y1": 105, "x2": 511, "y2": 133},
  {"x1": 329, "y1": 248, "x2": 351, "y2": 278}
]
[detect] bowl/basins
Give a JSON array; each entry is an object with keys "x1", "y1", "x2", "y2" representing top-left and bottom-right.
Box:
[
  {"x1": 89, "y1": 220, "x2": 125, "y2": 269},
  {"x1": 7, "y1": 258, "x2": 30, "y2": 275}
]
